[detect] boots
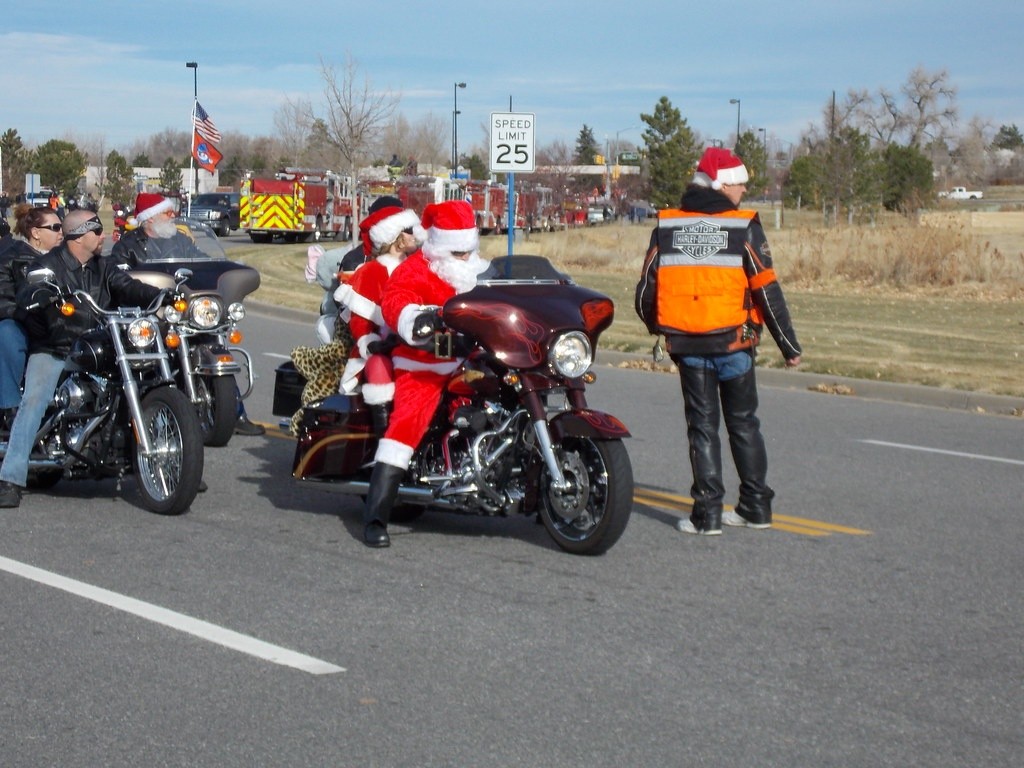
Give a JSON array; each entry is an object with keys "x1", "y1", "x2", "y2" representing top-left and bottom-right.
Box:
[
  {"x1": 364, "y1": 438, "x2": 414, "y2": 546},
  {"x1": 363, "y1": 383, "x2": 396, "y2": 435}
]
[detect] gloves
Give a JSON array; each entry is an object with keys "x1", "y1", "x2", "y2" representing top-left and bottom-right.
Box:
[
  {"x1": 32, "y1": 290, "x2": 59, "y2": 308},
  {"x1": 367, "y1": 334, "x2": 400, "y2": 354},
  {"x1": 413, "y1": 312, "x2": 447, "y2": 337},
  {"x1": 151, "y1": 287, "x2": 178, "y2": 305}
]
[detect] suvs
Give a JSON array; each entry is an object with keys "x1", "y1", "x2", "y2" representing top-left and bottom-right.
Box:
[{"x1": 180, "y1": 191, "x2": 239, "y2": 237}]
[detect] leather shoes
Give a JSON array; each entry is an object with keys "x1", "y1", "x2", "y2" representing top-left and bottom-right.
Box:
[{"x1": 233, "y1": 412, "x2": 266, "y2": 435}]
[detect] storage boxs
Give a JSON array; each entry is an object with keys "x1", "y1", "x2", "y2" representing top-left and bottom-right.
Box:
[
  {"x1": 272, "y1": 361, "x2": 308, "y2": 417},
  {"x1": 291, "y1": 396, "x2": 374, "y2": 478}
]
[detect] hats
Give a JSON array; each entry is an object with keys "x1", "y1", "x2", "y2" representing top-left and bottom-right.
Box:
[
  {"x1": 421, "y1": 201, "x2": 477, "y2": 252},
  {"x1": 358, "y1": 205, "x2": 421, "y2": 256},
  {"x1": 693, "y1": 147, "x2": 749, "y2": 190},
  {"x1": 130, "y1": 194, "x2": 172, "y2": 226}
]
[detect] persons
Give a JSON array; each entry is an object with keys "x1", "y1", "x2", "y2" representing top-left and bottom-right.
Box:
[
  {"x1": 634, "y1": 149, "x2": 802, "y2": 536},
  {"x1": 408, "y1": 156, "x2": 417, "y2": 175},
  {"x1": 390, "y1": 154, "x2": 404, "y2": 166},
  {"x1": 314, "y1": 196, "x2": 490, "y2": 546},
  {"x1": 112, "y1": 192, "x2": 266, "y2": 436},
  {"x1": 0, "y1": 187, "x2": 183, "y2": 507}
]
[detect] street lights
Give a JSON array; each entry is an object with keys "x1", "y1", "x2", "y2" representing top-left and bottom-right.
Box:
[
  {"x1": 614, "y1": 125, "x2": 641, "y2": 180},
  {"x1": 454, "y1": 81, "x2": 467, "y2": 178},
  {"x1": 186, "y1": 62, "x2": 199, "y2": 197},
  {"x1": 759, "y1": 128, "x2": 767, "y2": 156},
  {"x1": 729, "y1": 99, "x2": 740, "y2": 144},
  {"x1": 452, "y1": 111, "x2": 462, "y2": 173}
]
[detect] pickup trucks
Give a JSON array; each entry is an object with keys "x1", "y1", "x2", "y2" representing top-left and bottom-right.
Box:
[{"x1": 938, "y1": 185, "x2": 984, "y2": 201}]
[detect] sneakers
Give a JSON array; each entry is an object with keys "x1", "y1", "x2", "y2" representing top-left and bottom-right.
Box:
[
  {"x1": 721, "y1": 510, "x2": 771, "y2": 529},
  {"x1": 678, "y1": 518, "x2": 722, "y2": 534}
]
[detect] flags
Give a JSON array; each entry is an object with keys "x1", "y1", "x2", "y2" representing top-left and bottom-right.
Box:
[
  {"x1": 192, "y1": 129, "x2": 224, "y2": 176},
  {"x1": 193, "y1": 102, "x2": 227, "y2": 143}
]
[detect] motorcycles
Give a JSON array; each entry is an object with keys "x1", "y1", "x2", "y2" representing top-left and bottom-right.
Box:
[
  {"x1": 0, "y1": 204, "x2": 262, "y2": 517},
  {"x1": 271, "y1": 255, "x2": 636, "y2": 558}
]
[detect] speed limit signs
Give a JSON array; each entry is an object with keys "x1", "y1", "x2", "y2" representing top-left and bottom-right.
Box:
[{"x1": 491, "y1": 112, "x2": 536, "y2": 174}]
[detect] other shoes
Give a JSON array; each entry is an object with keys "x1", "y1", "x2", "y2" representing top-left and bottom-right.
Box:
[
  {"x1": 4, "y1": 407, "x2": 18, "y2": 431},
  {"x1": 0, "y1": 480, "x2": 22, "y2": 506},
  {"x1": 198, "y1": 480, "x2": 208, "y2": 490}
]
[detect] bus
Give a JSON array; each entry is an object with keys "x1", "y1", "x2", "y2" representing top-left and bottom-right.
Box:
[{"x1": 363, "y1": 173, "x2": 666, "y2": 237}]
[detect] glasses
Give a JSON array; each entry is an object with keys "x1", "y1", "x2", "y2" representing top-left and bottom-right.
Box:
[
  {"x1": 36, "y1": 223, "x2": 62, "y2": 231},
  {"x1": 89, "y1": 226, "x2": 103, "y2": 236},
  {"x1": 401, "y1": 227, "x2": 414, "y2": 234},
  {"x1": 161, "y1": 211, "x2": 176, "y2": 218}
]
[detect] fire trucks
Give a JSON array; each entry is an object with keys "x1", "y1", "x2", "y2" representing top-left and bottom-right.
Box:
[{"x1": 237, "y1": 167, "x2": 367, "y2": 244}]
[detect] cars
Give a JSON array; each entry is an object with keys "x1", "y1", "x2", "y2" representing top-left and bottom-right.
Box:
[{"x1": 25, "y1": 192, "x2": 55, "y2": 208}]
